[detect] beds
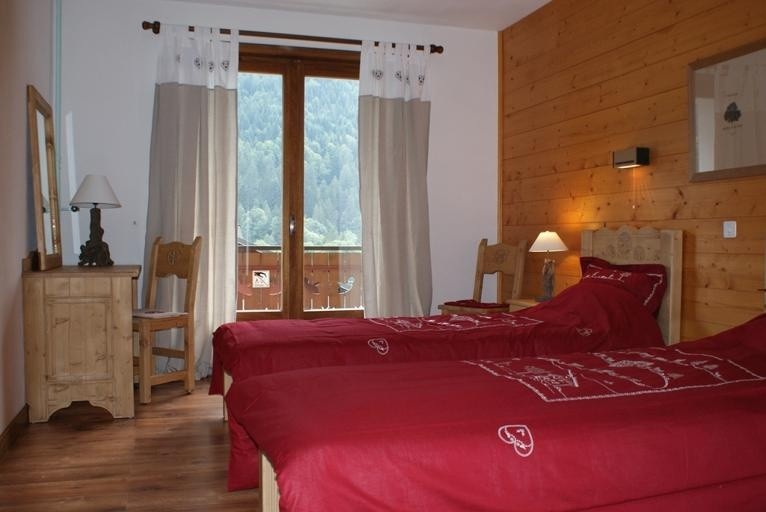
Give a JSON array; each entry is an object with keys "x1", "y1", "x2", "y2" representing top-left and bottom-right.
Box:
[
  {"x1": 224, "y1": 335, "x2": 766, "y2": 512},
  {"x1": 201, "y1": 230, "x2": 684, "y2": 420}
]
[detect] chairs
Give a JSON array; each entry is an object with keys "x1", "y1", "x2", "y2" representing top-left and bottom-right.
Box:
[
  {"x1": 133, "y1": 233, "x2": 201, "y2": 404},
  {"x1": 438, "y1": 237, "x2": 527, "y2": 314}
]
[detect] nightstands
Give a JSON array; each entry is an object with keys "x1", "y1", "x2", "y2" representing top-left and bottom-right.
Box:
[{"x1": 504, "y1": 297, "x2": 543, "y2": 320}]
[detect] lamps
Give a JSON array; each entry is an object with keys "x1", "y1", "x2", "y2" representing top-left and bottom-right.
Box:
[
  {"x1": 529, "y1": 232, "x2": 568, "y2": 302},
  {"x1": 612, "y1": 144, "x2": 649, "y2": 173},
  {"x1": 69, "y1": 173, "x2": 122, "y2": 268}
]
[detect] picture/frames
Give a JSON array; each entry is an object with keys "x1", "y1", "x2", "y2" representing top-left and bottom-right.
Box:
[{"x1": 686, "y1": 40, "x2": 766, "y2": 183}]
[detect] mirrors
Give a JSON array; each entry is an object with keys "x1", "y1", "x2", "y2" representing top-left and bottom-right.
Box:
[{"x1": 26, "y1": 84, "x2": 63, "y2": 270}]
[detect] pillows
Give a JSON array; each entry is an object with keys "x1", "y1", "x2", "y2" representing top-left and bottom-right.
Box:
[{"x1": 576, "y1": 256, "x2": 664, "y2": 322}]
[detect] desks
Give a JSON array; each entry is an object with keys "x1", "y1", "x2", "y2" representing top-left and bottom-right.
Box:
[{"x1": 21, "y1": 253, "x2": 142, "y2": 423}]
